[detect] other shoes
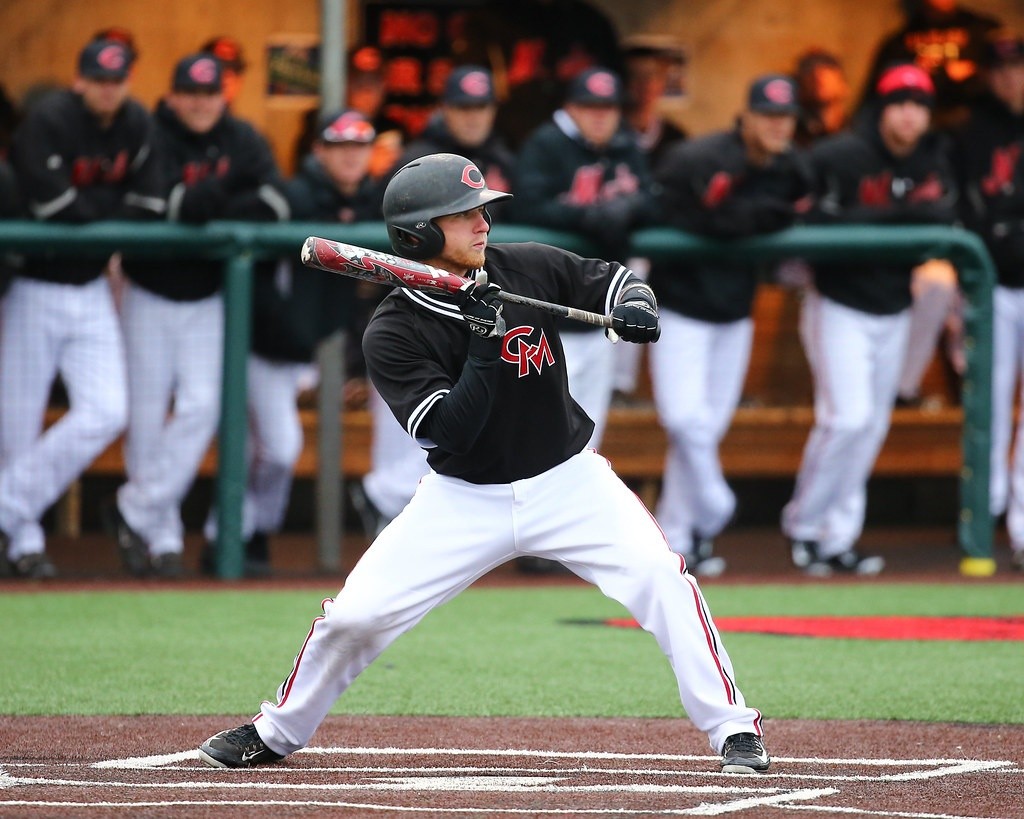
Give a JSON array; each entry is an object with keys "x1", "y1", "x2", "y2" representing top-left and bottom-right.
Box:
[
  {"x1": 148, "y1": 551, "x2": 183, "y2": 581},
  {"x1": 246, "y1": 532, "x2": 277, "y2": 578},
  {"x1": 831, "y1": 548, "x2": 886, "y2": 575},
  {"x1": 11, "y1": 548, "x2": 54, "y2": 581},
  {"x1": 691, "y1": 530, "x2": 728, "y2": 581},
  {"x1": 789, "y1": 538, "x2": 839, "y2": 578},
  {"x1": 115, "y1": 529, "x2": 154, "y2": 581}
]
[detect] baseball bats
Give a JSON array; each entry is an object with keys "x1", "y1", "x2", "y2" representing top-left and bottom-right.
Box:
[{"x1": 300, "y1": 235, "x2": 661, "y2": 344}]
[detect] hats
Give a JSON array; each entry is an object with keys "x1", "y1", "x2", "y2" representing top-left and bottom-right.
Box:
[
  {"x1": 747, "y1": 74, "x2": 802, "y2": 115},
  {"x1": 170, "y1": 53, "x2": 224, "y2": 95},
  {"x1": 444, "y1": 64, "x2": 498, "y2": 112},
  {"x1": 565, "y1": 65, "x2": 632, "y2": 113},
  {"x1": 315, "y1": 109, "x2": 378, "y2": 147},
  {"x1": 76, "y1": 37, "x2": 135, "y2": 82},
  {"x1": 875, "y1": 66, "x2": 933, "y2": 105}
]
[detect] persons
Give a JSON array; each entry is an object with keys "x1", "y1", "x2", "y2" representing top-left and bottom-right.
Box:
[
  {"x1": 202, "y1": 153, "x2": 774, "y2": 772},
  {"x1": 0, "y1": 0, "x2": 1023, "y2": 579}
]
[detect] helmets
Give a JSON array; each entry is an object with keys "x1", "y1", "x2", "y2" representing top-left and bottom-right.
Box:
[{"x1": 383, "y1": 151, "x2": 516, "y2": 260}]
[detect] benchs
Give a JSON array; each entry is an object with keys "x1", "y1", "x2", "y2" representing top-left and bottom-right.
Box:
[{"x1": 44, "y1": 282, "x2": 1023, "y2": 541}]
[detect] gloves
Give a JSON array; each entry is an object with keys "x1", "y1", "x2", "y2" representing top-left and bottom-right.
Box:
[
  {"x1": 171, "y1": 184, "x2": 225, "y2": 226},
  {"x1": 23, "y1": 160, "x2": 67, "y2": 208},
  {"x1": 457, "y1": 277, "x2": 509, "y2": 359},
  {"x1": 604, "y1": 298, "x2": 657, "y2": 346}
]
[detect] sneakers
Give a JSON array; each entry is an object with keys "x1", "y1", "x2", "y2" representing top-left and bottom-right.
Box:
[
  {"x1": 201, "y1": 720, "x2": 288, "y2": 773},
  {"x1": 718, "y1": 733, "x2": 772, "y2": 776}
]
